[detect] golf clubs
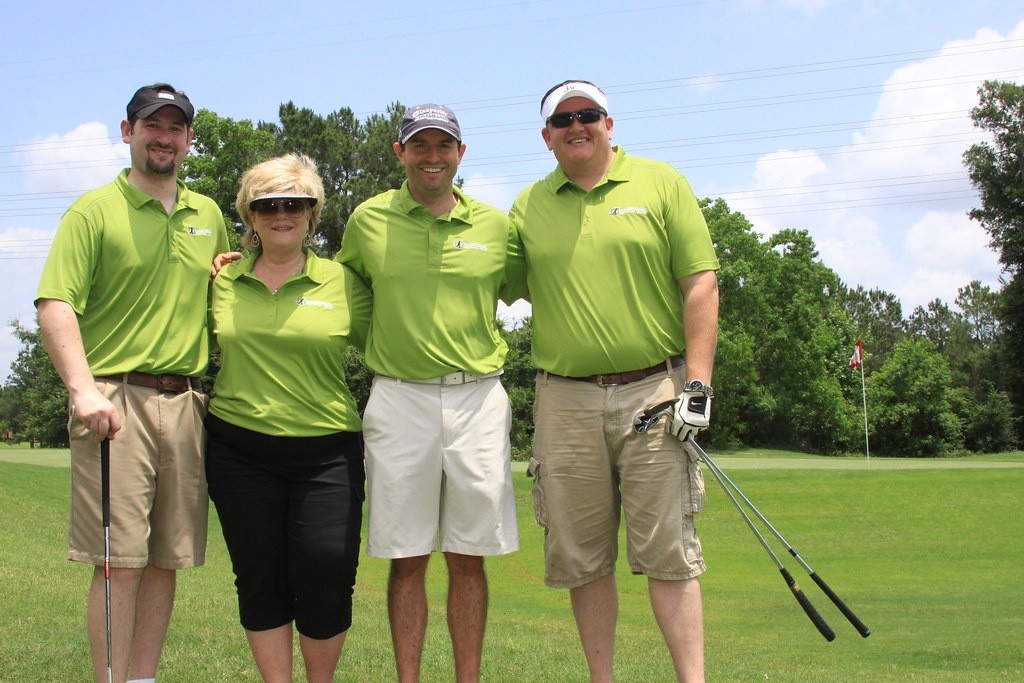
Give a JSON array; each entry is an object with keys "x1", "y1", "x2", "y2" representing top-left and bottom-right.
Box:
[
  {"x1": 631, "y1": 396, "x2": 874, "y2": 643},
  {"x1": 99, "y1": 439, "x2": 117, "y2": 681}
]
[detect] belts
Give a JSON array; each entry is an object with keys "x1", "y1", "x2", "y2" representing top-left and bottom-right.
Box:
[
  {"x1": 536, "y1": 354, "x2": 686, "y2": 385},
  {"x1": 406, "y1": 366, "x2": 504, "y2": 386},
  {"x1": 98, "y1": 371, "x2": 202, "y2": 392}
]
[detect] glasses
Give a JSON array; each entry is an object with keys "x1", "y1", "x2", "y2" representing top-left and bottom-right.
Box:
[
  {"x1": 250, "y1": 199, "x2": 312, "y2": 221},
  {"x1": 546, "y1": 107, "x2": 607, "y2": 128}
]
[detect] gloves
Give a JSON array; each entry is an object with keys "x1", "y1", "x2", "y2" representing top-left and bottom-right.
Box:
[{"x1": 664, "y1": 392, "x2": 714, "y2": 442}]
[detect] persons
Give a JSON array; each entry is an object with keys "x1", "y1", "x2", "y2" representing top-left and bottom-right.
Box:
[
  {"x1": 208, "y1": 152, "x2": 374, "y2": 683},
  {"x1": 33, "y1": 84, "x2": 230, "y2": 683},
  {"x1": 507, "y1": 80, "x2": 721, "y2": 681},
  {"x1": 211, "y1": 104, "x2": 531, "y2": 683}
]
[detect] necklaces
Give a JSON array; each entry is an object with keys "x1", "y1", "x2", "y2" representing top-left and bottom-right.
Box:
[{"x1": 253, "y1": 258, "x2": 304, "y2": 292}]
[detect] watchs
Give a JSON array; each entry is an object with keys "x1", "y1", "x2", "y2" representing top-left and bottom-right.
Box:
[{"x1": 684, "y1": 379, "x2": 713, "y2": 395}]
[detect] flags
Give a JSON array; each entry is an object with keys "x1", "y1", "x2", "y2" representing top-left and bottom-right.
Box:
[{"x1": 849, "y1": 340, "x2": 862, "y2": 369}]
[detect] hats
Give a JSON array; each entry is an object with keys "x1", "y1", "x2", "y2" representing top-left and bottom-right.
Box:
[
  {"x1": 248, "y1": 191, "x2": 318, "y2": 202},
  {"x1": 399, "y1": 103, "x2": 462, "y2": 145},
  {"x1": 541, "y1": 82, "x2": 608, "y2": 124},
  {"x1": 127, "y1": 89, "x2": 194, "y2": 128}
]
